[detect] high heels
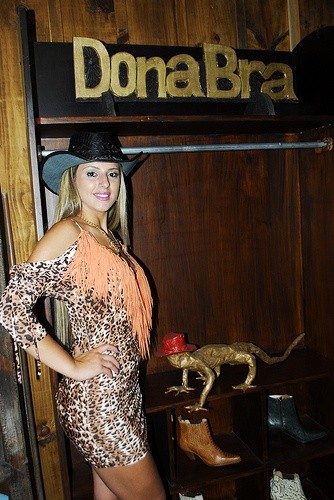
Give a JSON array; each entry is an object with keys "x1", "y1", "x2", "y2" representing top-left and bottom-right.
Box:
[
  {"x1": 178, "y1": 417, "x2": 241, "y2": 466},
  {"x1": 268, "y1": 394, "x2": 328, "y2": 445}
]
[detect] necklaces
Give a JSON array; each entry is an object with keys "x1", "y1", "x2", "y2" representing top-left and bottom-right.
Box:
[{"x1": 77, "y1": 213, "x2": 122, "y2": 253}]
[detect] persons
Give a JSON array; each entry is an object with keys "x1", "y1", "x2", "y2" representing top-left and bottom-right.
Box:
[{"x1": 0, "y1": 131, "x2": 166, "y2": 500}]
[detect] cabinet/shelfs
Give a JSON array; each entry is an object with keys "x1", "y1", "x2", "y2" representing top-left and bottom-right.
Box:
[{"x1": 143, "y1": 349, "x2": 334, "y2": 500}]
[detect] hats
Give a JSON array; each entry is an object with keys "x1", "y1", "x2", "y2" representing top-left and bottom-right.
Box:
[{"x1": 43, "y1": 131, "x2": 143, "y2": 197}]
